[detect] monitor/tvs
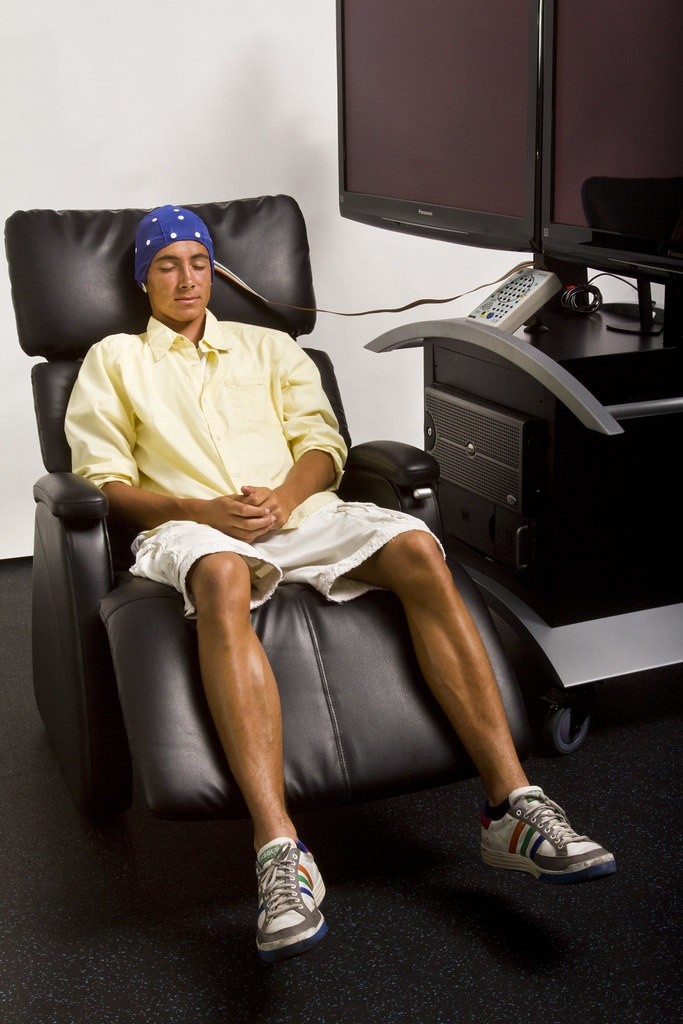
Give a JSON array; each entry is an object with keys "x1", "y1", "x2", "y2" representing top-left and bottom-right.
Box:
[
  {"x1": 336, "y1": 0, "x2": 542, "y2": 255},
  {"x1": 538, "y1": 0, "x2": 683, "y2": 286}
]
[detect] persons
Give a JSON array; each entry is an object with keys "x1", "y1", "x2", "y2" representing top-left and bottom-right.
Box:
[{"x1": 63, "y1": 204, "x2": 617, "y2": 961}]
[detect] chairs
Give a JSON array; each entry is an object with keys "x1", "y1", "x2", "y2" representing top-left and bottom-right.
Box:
[{"x1": 5, "y1": 194, "x2": 595, "y2": 829}]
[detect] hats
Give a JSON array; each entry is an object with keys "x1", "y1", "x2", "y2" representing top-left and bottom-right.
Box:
[{"x1": 134, "y1": 206, "x2": 215, "y2": 287}]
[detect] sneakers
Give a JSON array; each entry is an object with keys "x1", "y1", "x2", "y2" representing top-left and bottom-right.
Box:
[
  {"x1": 252, "y1": 836, "x2": 329, "y2": 964},
  {"x1": 480, "y1": 786, "x2": 617, "y2": 886}
]
